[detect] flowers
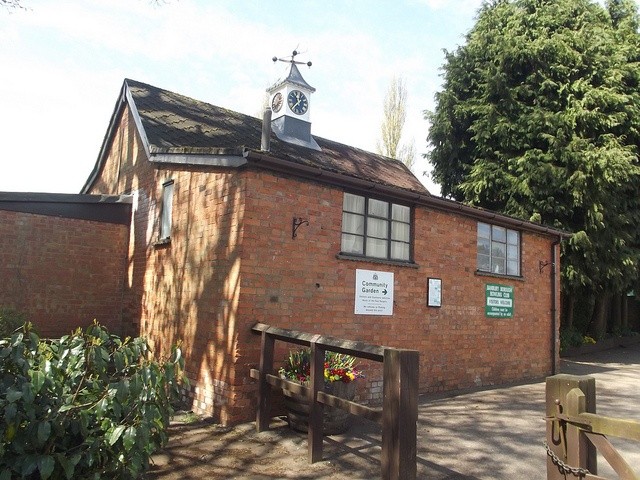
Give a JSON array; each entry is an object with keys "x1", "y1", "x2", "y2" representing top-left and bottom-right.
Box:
[{"x1": 281, "y1": 345, "x2": 366, "y2": 386}]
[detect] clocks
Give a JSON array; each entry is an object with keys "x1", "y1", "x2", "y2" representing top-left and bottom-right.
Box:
[
  {"x1": 271, "y1": 92, "x2": 283, "y2": 111},
  {"x1": 288, "y1": 90, "x2": 308, "y2": 115}
]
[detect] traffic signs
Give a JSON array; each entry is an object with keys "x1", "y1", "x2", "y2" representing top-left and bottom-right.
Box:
[{"x1": 354, "y1": 269, "x2": 394, "y2": 316}]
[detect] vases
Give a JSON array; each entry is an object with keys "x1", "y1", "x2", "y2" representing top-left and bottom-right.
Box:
[{"x1": 279, "y1": 370, "x2": 356, "y2": 435}]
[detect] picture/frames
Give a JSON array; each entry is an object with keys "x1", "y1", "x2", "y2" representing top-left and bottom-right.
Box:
[{"x1": 428, "y1": 277, "x2": 442, "y2": 306}]
